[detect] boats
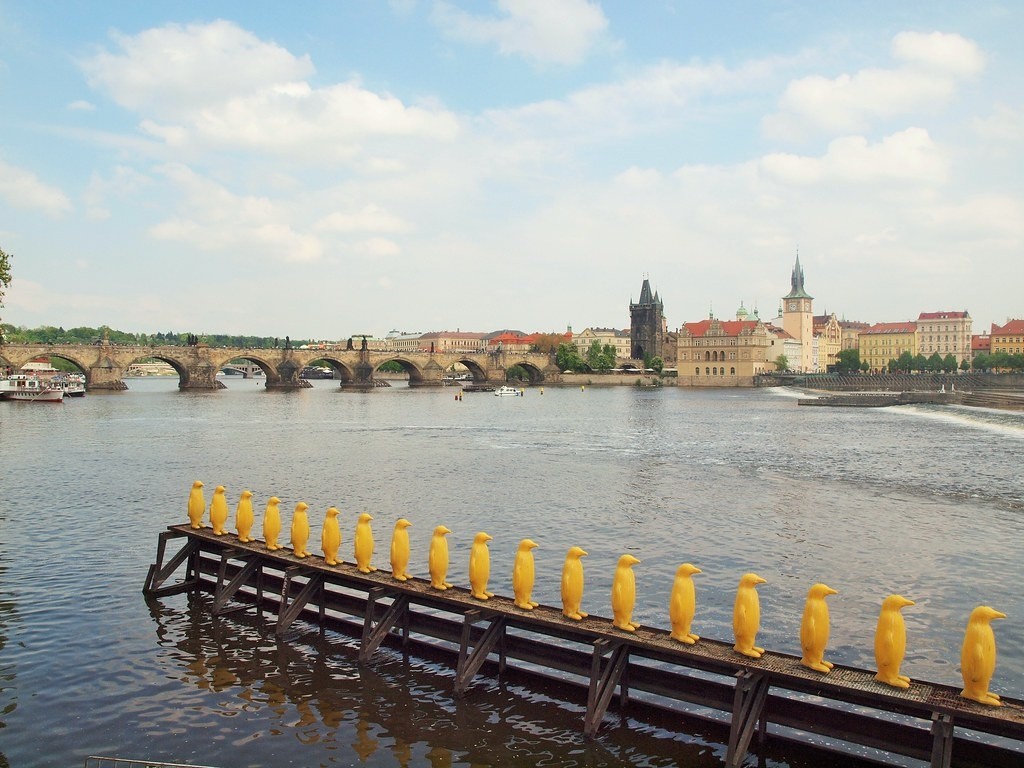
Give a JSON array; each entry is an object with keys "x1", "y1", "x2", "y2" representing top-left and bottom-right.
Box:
[
  {"x1": 45, "y1": 371, "x2": 86, "y2": 397},
  {"x1": 495, "y1": 385, "x2": 521, "y2": 396},
  {"x1": 462, "y1": 383, "x2": 496, "y2": 392},
  {"x1": 0, "y1": 369, "x2": 65, "y2": 402}
]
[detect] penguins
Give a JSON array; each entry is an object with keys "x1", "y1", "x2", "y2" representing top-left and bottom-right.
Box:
[
  {"x1": 957, "y1": 605, "x2": 1006, "y2": 708},
  {"x1": 798, "y1": 583, "x2": 834, "y2": 673},
  {"x1": 872, "y1": 594, "x2": 916, "y2": 690},
  {"x1": 186, "y1": 480, "x2": 769, "y2": 660}
]
